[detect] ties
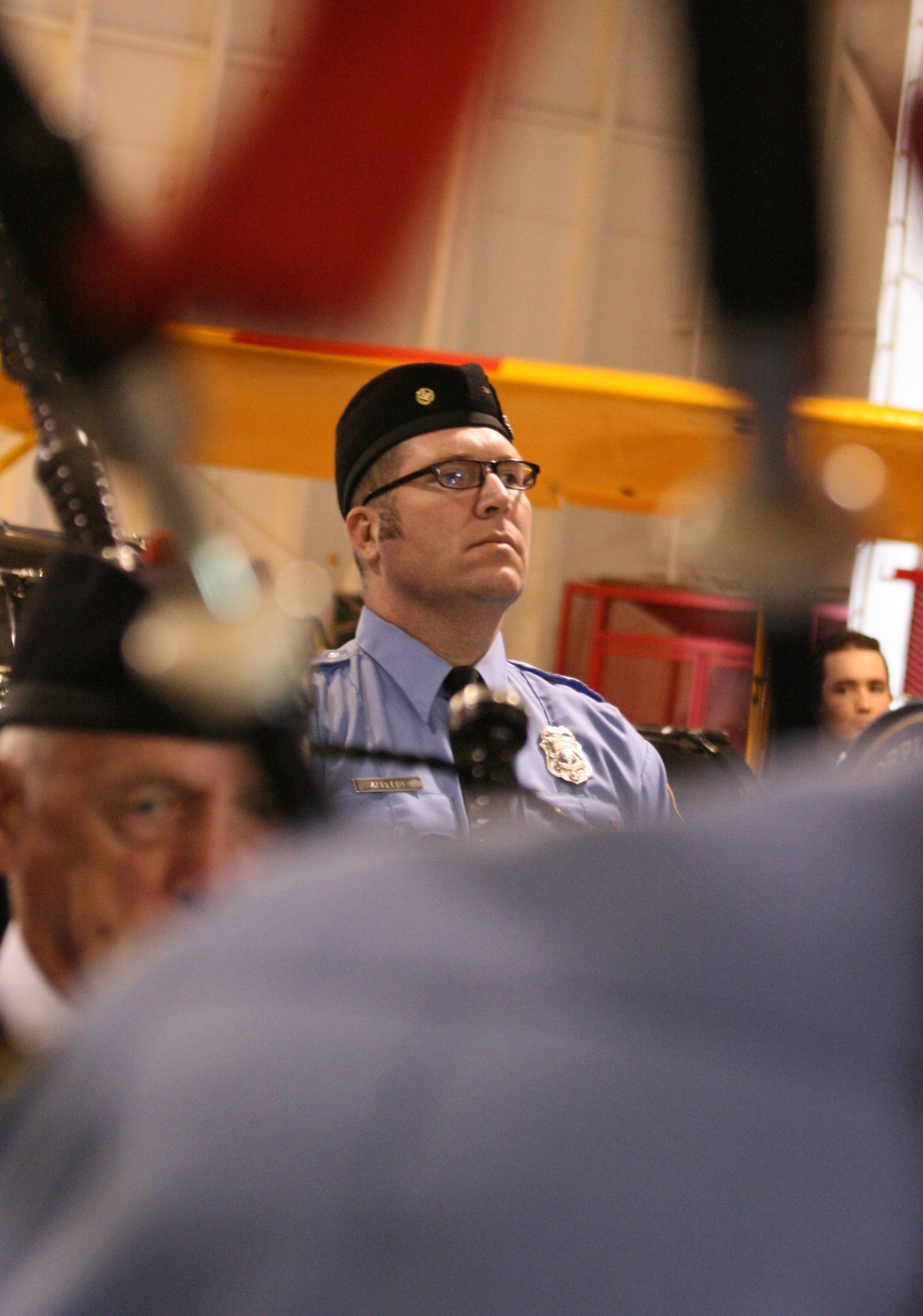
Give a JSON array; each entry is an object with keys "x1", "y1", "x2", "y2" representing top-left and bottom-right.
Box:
[{"x1": 446, "y1": 664, "x2": 512, "y2": 834}]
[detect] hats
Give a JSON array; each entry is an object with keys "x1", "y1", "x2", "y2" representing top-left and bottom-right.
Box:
[
  {"x1": 2, "y1": 544, "x2": 261, "y2": 743},
  {"x1": 335, "y1": 362, "x2": 514, "y2": 518}
]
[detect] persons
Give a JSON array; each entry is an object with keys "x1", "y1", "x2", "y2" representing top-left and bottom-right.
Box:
[
  {"x1": 300, "y1": 362, "x2": 679, "y2": 832},
  {"x1": 0, "y1": 707, "x2": 923, "y2": 1316},
  {"x1": 0, "y1": 546, "x2": 284, "y2": 1092},
  {"x1": 818, "y1": 631, "x2": 891, "y2": 752}
]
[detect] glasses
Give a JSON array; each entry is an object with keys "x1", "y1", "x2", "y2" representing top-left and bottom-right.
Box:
[{"x1": 359, "y1": 459, "x2": 539, "y2": 505}]
